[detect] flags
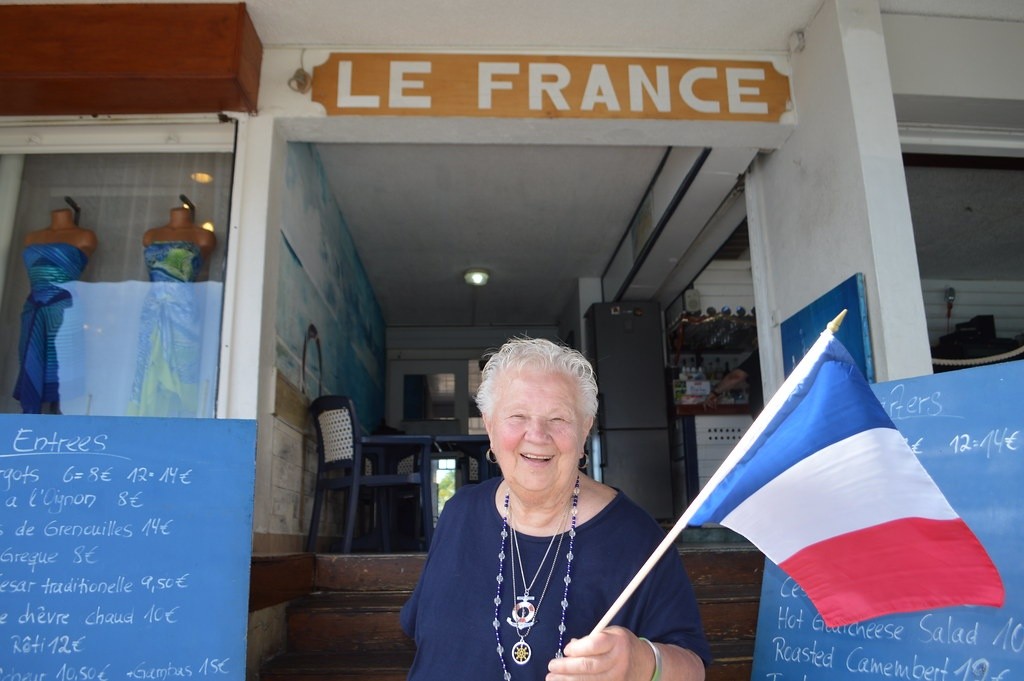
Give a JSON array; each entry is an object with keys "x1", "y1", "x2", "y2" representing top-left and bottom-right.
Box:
[{"x1": 688, "y1": 336, "x2": 1005, "y2": 627}]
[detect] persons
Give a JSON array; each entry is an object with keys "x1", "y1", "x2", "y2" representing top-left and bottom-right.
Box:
[
  {"x1": 127, "y1": 208, "x2": 216, "y2": 419},
  {"x1": 13, "y1": 209, "x2": 99, "y2": 415},
  {"x1": 703, "y1": 348, "x2": 764, "y2": 421},
  {"x1": 398, "y1": 337, "x2": 710, "y2": 681}
]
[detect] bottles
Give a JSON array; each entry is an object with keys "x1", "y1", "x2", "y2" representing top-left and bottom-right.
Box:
[{"x1": 668, "y1": 356, "x2": 731, "y2": 383}]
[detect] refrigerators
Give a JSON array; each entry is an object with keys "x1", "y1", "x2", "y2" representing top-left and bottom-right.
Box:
[{"x1": 583, "y1": 301, "x2": 675, "y2": 525}]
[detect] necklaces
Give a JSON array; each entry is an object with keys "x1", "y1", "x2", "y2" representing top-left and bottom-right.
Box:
[{"x1": 493, "y1": 476, "x2": 580, "y2": 681}]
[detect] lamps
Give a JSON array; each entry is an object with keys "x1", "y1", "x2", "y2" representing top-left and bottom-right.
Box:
[{"x1": 464, "y1": 266, "x2": 491, "y2": 289}]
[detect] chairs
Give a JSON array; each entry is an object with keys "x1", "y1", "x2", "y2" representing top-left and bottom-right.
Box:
[{"x1": 297, "y1": 394, "x2": 428, "y2": 552}]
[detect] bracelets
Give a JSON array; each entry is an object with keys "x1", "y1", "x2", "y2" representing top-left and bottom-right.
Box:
[
  {"x1": 711, "y1": 390, "x2": 720, "y2": 397},
  {"x1": 639, "y1": 637, "x2": 662, "y2": 681}
]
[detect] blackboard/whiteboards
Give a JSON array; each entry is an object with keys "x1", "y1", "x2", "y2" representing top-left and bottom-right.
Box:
[
  {"x1": 0, "y1": 412, "x2": 258, "y2": 681},
  {"x1": 779, "y1": 273, "x2": 877, "y2": 386},
  {"x1": 750, "y1": 358, "x2": 1024, "y2": 681}
]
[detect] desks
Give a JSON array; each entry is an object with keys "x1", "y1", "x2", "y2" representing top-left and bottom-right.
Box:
[{"x1": 358, "y1": 435, "x2": 497, "y2": 547}]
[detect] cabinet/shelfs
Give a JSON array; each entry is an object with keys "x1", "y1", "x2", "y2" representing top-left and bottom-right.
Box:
[{"x1": 675, "y1": 403, "x2": 761, "y2": 529}]
